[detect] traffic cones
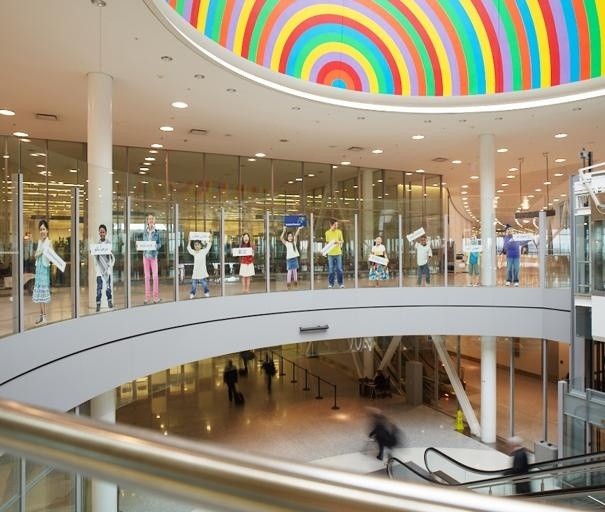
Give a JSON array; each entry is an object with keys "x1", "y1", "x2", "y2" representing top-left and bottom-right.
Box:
[{"x1": 454, "y1": 410, "x2": 464, "y2": 430}]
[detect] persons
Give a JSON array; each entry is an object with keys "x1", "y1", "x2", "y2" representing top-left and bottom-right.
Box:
[
  {"x1": 230, "y1": 232, "x2": 256, "y2": 293},
  {"x1": 461, "y1": 229, "x2": 483, "y2": 286},
  {"x1": 93, "y1": 224, "x2": 116, "y2": 312},
  {"x1": 313, "y1": 256, "x2": 328, "y2": 266},
  {"x1": 279, "y1": 226, "x2": 303, "y2": 289},
  {"x1": 366, "y1": 407, "x2": 407, "y2": 461},
  {"x1": 368, "y1": 235, "x2": 389, "y2": 287},
  {"x1": 320, "y1": 219, "x2": 344, "y2": 288},
  {"x1": 186, "y1": 233, "x2": 213, "y2": 299},
  {"x1": 135, "y1": 213, "x2": 162, "y2": 304},
  {"x1": 223, "y1": 351, "x2": 277, "y2": 403},
  {"x1": 32, "y1": 220, "x2": 53, "y2": 325},
  {"x1": 372, "y1": 370, "x2": 387, "y2": 399},
  {"x1": 407, "y1": 232, "x2": 433, "y2": 287},
  {"x1": 502, "y1": 223, "x2": 531, "y2": 288},
  {"x1": 502, "y1": 436, "x2": 531, "y2": 494}
]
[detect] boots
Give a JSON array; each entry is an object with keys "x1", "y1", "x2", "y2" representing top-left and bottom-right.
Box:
[{"x1": 287, "y1": 281, "x2": 297, "y2": 288}]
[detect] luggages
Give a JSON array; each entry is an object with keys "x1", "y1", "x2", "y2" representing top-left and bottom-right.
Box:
[{"x1": 233, "y1": 383, "x2": 245, "y2": 406}]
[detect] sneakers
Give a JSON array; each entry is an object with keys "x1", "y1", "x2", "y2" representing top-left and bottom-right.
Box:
[
  {"x1": 339, "y1": 284, "x2": 344, "y2": 288},
  {"x1": 188, "y1": 292, "x2": 194, "y2": 298},
  {"x1": 33, "y1": 312, "x2": 47, "y2": 325},
  {"x1": 107, "y1": 301, "x2": 112, "y2": 307},
  {"x1": 467, "y1": 280, "x2": 518, "y2": 287},
  {"x1": 328, "y1": 283, "x2": 332, "y2": 287},
  {"x1": 205, "y1": 292, "x2": 208, "y2": 297},
  {"x1": 95, "y1": 305, "x2": 99, "y2": 311}
]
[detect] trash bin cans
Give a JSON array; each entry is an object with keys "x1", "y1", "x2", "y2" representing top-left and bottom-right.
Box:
[
  {"x1": 534, "y1": 440, "x2": 558, "y2": 470},
  {"x1": 358, "y1": 378, "x2": 370, "y2": 396},
  {"x1": 454, "y1": 253, "x2": 467, "y2": 273}
]
